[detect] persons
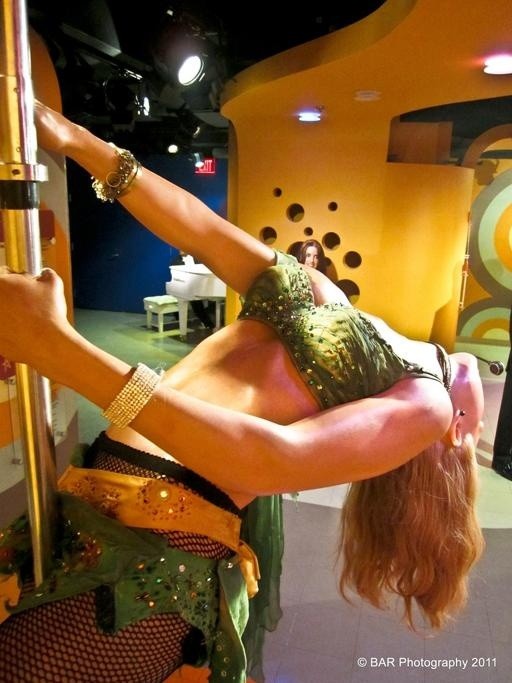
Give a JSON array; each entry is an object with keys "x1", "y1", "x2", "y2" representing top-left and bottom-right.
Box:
[
  {"x1": 101, "y1": 360, "x2": 163, "y2": 431},
  {"x1": 1, "y1": 100, "x2": 485, "y2": 683},
  {"x1": 295, "y1": 238, "x2": 325, "y2": 271}
]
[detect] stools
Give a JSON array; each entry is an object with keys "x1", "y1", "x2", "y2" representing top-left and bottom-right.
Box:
[{"x1": 144, "y1": 295, "x2": 179, "y2": 333}]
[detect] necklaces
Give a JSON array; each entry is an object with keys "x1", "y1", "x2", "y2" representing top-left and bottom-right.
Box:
[{"x1": 428, "y1": 342, "x2": 452, "y2": 391}]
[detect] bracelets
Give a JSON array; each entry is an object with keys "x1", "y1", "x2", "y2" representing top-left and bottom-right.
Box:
[{"x1": 91, "y1": 142, "x2": 140, "y2": 203}]
